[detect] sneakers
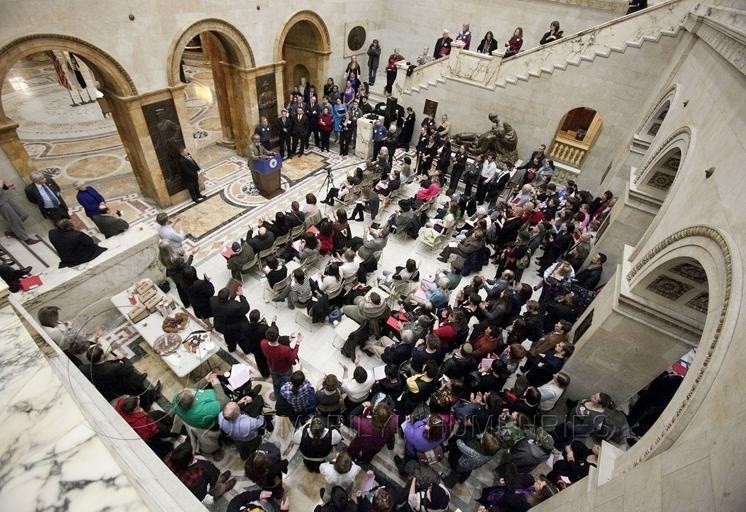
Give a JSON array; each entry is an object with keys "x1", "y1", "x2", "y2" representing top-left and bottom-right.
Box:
[
  {"x1": 271, "y1": 297, "x2": 285, "y2": 302},
  {"x1": 437, "y1": 257, "x2": 447, "y2": 263}
]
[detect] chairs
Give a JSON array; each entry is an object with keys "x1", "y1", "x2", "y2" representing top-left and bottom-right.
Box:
[{"x1": 171, "y1": 167, "x2": 472, "y2": 452}]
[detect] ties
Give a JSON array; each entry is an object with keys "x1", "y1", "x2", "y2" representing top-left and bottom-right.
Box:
[{"x1": 42, "y1": 184, "x2": 60, "y2": 209}]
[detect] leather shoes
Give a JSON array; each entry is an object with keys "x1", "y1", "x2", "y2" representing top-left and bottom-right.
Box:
[
  {"x1": 394, "y1": 455, "x2": 401, "y2": 465},
  {"x1": 26, "y1": 238, "x2": 41, "y2": 245},
  {"x1": 221, "y1": 470, "x2": 231, "y2": 483},
  {"x1": 327, "y1": 202, "x2": 334, "y2": 206},
  {"x1": 347, "y1": 217, "x2": 355, "y2": 220},
  {"x1": 226, "y1": 478, "x2": 236, "y2": 491},
  {"x1": 153, "y1": 379, "x2": 161, "y2": 402},
  {"x1": 355, "y1": 219, "x2": 364, "y2": 221},
  {"x1": 4, "y1": 232, "x2": 18, "y2": 238},
  {"x1": 320, "y1": 200, "x2": 329, "y2": 204},
  {"x1": 253, "y1": 384, "x2": 262, "y2": 395}
]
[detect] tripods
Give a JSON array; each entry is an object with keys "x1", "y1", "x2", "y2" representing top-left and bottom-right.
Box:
[{"x1": 316, "y1": 175, "x2": 337, "y2": 213}]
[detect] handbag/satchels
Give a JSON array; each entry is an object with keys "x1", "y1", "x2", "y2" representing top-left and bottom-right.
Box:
[
  {"x1": 386, "y1": 431, "x2": 395, "y2": 450},
  {"x1": 18, "y1": 273, "x2": 41, "y2": 291}
]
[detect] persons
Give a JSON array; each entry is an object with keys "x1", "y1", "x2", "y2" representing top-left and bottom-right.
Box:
[{"x1": 0, "y1": 0, "x2": 685, "y2": 511}]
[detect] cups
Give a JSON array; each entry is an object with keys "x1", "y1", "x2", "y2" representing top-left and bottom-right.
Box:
[{"x1": 128, "y1": 295, "x2": 137, "y2": 305}]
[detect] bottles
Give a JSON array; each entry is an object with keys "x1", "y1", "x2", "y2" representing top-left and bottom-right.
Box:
[{"x1": 159, "y1": 303, "x2": 173, "y2": 318}]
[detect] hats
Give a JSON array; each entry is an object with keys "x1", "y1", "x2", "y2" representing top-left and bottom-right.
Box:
[
  {"x1": 536, "y1": 427, "x2": 555, "y2": 450},
  {"x1": 231, "y1": 241, "x2": 241, "y2": 252},
  {"x1": 451, "y1": 261, "x2": 464, "y2": 271}
]
